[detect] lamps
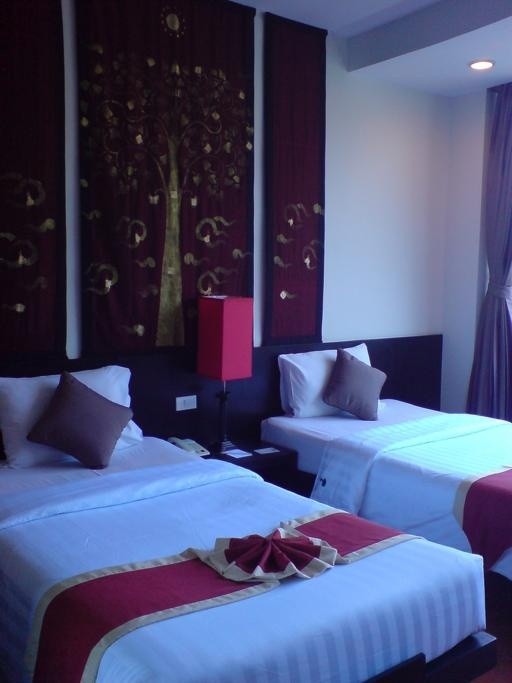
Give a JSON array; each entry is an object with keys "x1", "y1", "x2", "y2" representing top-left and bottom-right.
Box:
[{"x1": 196, "y1": 294, "x2": 251, "y2": 457}]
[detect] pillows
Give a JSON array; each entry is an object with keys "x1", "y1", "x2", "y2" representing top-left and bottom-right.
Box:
[
  {"x1": 322, "y1": 347, "x2": 388, "y2": 421},
  {"x1": 277, "y1": 341, "x2": 372, "y2": 420},
  {"x1": 1, "y1": 364, "x2": 145, "y2": 469},
  {"x1": 26, "y1": 373, "x2": 135, "y2": 468}
]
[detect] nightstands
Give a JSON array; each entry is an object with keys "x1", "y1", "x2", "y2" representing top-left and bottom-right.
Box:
[{"x1": 206, "y1": 440, "x2": 299, "y2": 494}]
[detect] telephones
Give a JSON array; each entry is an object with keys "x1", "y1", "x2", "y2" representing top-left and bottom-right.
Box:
[{"x1": 168, "y1": 437, "x2": 210, "y2": 457}]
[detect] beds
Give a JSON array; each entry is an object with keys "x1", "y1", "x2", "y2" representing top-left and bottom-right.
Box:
[
  {"x1": 223, "y1": 332, "x2": 512, "y2": 612},
  {"x1": 1, "y1": 344, "x2": 498, "y2": 683}
]
